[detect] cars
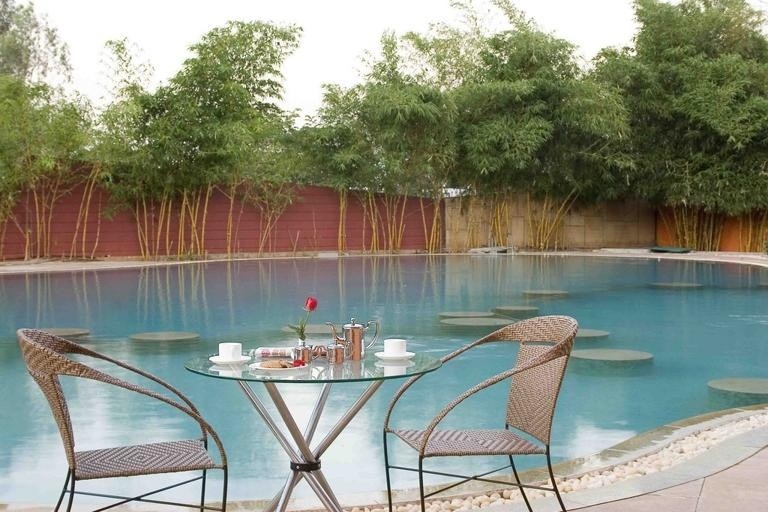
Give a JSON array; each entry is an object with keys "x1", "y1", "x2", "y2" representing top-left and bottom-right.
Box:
[
  {"x1": 317, "y1": 343, "x2": 345, "y2": 364},
  {"x1": 218, "y1": 370, "x2": 242, "y2": 379},
  {"x1": 218, "y1": 342, "x2": 242, "y2": 359},
  {"x1": 384, "y1": 366, "x2": 407, "y2": 377},
  {"x1": 292, "y1": 341, "x2": 322, "y2": 363},
  {"x1": 383, "y1": 339, "x2": 406, "y2": 355}
]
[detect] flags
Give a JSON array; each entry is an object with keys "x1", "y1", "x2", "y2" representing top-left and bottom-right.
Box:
[{"x1": 289, "y1": 338, "x2": 313, "y2": 360}]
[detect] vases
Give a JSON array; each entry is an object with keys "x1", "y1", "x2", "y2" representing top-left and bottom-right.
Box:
[{"x1": 287, "y1": 297, "x2": 319, "y2": 341}]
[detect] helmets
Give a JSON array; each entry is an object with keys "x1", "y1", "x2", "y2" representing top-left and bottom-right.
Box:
[
  {"x1": 248, "y1": 360, "x2": 309, "y2": 371},
  {"x1": 374, "y1": 360, "x2": 415, "y2": 367},
  {"x1": 209, "y1": 364, "x2": 252, "y2": 372},
  {"x1": 208, "y1": 356, "x2": 252, "y2": 365},
  {"x1": 373, "y1": 351, "x2": 416, "y2": 362},
  {"x1": 249, "y1": 370, "x2": 308, "y2": 378}
]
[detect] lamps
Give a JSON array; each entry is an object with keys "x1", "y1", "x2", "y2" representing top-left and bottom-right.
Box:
[
  {"x1": 15, "y1": 326, "x2": 229, "y2": 512},
  {"x1": 382, "y1": 315, "x2": 578, "y2": 511}
]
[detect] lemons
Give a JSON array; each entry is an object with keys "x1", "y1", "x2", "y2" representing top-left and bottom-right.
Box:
[{"x1": 325, "y1": 317, "x2": 379, "y2": 361}]
